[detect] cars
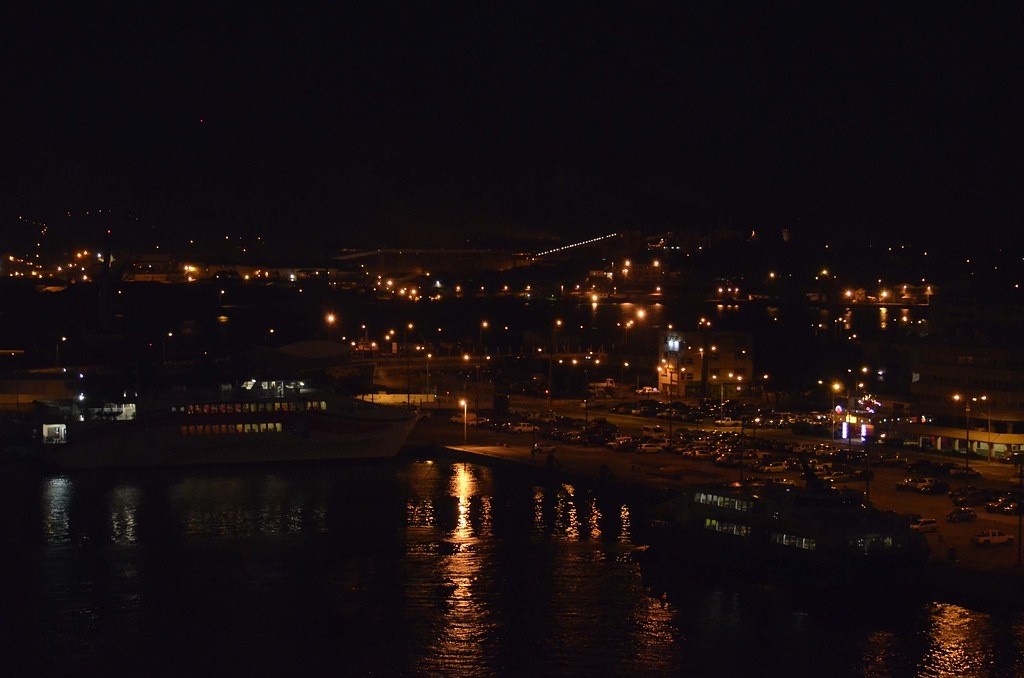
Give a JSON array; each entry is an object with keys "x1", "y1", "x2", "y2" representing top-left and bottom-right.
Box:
[{"x1": 448, "y1": 374, "x2": 1024, "y2": 549}]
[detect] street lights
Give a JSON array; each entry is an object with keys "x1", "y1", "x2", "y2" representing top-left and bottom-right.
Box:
[
  {"x1": 950, "y1": 391, "x2": 989, "y2": 488},
  {"x1": 667, "y1": 367, "x2": 687, "y2": 448},
  {"x1": 830, "y1": 381, "x2": 844, "y2": 445},
  {"x1": 739, "y1": 415, "x2": 761, "y2": 481},
  {"x1": 458, "y1": 399, "x2": 469, "y2": 447}
]
